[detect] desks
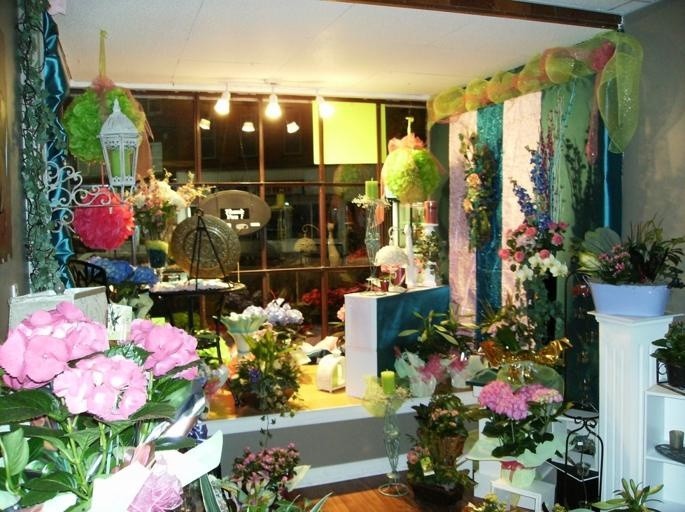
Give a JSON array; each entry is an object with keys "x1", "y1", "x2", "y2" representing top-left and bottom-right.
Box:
[{"x1": 146, "y1": 281, "x2": 247, "y2": 334}]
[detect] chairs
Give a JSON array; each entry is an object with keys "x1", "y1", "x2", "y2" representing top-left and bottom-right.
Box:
[{"x1": 68, "y1": 258, "x2": 173, "y2": 325}]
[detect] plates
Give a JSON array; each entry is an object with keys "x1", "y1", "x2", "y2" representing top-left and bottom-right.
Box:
[{"x1": 171, "y1": 214, "x2": 242, "y2": 279}]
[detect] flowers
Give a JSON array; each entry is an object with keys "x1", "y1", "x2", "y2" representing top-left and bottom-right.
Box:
[
  {"x1": 465, "y1": 378, "x2": 576, "y2": 488},
  {"x1": 129, "y1": 168, "x2": 216, "y2": 242},
  {"x1": 405, "y1": 395, "x2": 471, "y2": 488},
  {"x1": 478, "y1": 126, "x2": 576, "y2": 372},
  {"x1": 580, "y1": 219, "x2": 684, "y2": 285}
]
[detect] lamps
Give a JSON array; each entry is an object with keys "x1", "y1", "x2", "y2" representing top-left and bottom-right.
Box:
[
  {"x1": 286, "y1": 117, "x2": 298, "y2": 134},
  {"x1": 98, "y1": 100, "x2": 143, "y2": 196},
  {"x1": 199, "y1": 111, "x2": 212, "y2": 130},
  {"x1": 241, "y1": 115, "x2": 255, "y2": 132},
  {"x1": 214, "y1": 82, "x2": 335, "y2": 120}
]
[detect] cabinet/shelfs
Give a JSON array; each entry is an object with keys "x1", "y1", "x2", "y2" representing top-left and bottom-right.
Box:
[{"x1": 643, "y1": 383, "x2": 684, "y2": 512}]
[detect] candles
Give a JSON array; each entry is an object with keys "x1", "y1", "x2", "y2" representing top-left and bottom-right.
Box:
[
  {"x1": 276, "y1": 191, "x2": 284, "y2": 203},
  {"x1": 380, "y1": 368, "x2": 395, "y2": 392},
  {"x1": 364, "y1": 178, "x2": 379, "y2": 200}
]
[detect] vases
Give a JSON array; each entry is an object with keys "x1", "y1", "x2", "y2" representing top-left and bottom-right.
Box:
[
  {"x1": 591, "y1": 283, "x2": 669, "y2": 316},
  {"x1": 144, "y1": 240, "x2": 168, "y2": 268},
  {"x1": 411, "y1": 474, "x2": 476, "y2": 511}
]
